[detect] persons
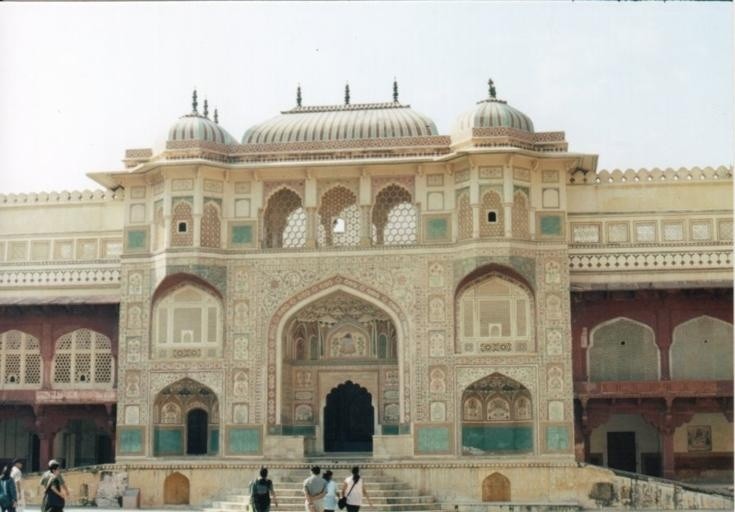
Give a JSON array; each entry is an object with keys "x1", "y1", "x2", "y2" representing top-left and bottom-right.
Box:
[
  {"x1": 0, "y1": 465, "x2": 17, "y2": 512},
  {"x1": 341, "y1": 466, "x2": 373, "y2": 512},
  {"x1": 40, "y1": 459, "x2": 70, "y2": 499},
  {"x1": 249, "y1": 467, "x2": 278, "y2": 512},
  {"x1": 9, "y1": 458, "x2": 26, "y2": 512},
  {"x1": 303, "y1": 466, "x2": 327, "y2": 512},
  {"x1": 43, "y1": 463, "x2": 65, "y2": 512},
  {"x1": 322, "y1": 470, "x2": 340, "y2": 512}
]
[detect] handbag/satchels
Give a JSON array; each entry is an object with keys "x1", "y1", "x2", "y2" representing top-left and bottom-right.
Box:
[{"x1": 339, "y1": 497, "x2": 346, "y2": 508}]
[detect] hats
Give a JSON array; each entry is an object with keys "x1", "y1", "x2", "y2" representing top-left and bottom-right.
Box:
[{"x1": 49, "y1": 460, "x2": 58, "y2": 467}]
